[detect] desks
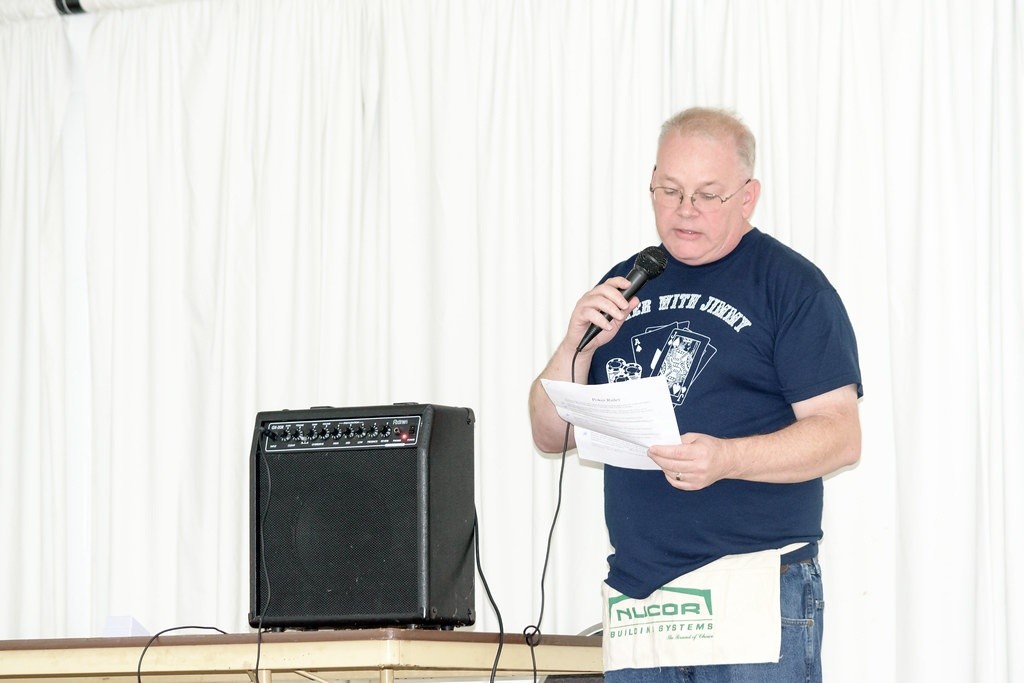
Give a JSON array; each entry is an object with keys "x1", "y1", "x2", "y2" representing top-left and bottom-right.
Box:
[{"x1": 0, "y1": 630, "x2": 604, "y2": 683}]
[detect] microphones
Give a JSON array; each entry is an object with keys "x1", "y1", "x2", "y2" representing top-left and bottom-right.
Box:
[{"x1": 575, "y1": 246, "x2": 669, "y2": 353}]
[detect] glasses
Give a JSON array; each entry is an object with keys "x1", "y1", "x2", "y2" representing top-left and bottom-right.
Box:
[{"x1": 650, "y1": 166, "x2": 751, "y2": 211}]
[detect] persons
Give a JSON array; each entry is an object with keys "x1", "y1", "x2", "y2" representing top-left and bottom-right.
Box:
[{"x1": 527, "y1": 108, "x2": 864, "y2": 683}]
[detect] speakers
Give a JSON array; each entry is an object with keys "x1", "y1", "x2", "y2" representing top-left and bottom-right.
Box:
[{"x1": 248, "y1": 402, "x2": 477, "y2": 630}]
